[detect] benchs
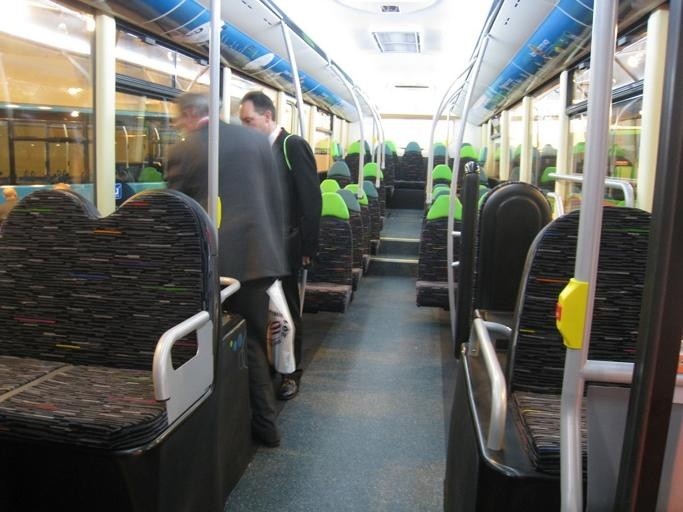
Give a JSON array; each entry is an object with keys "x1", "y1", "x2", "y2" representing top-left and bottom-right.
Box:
[{"x1": 1, "y1": 188, "x2": 218, "y2": 509}]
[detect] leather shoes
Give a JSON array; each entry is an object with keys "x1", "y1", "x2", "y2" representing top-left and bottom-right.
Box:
[
  {"x1": 251, "y1": 424, "x2": 280, "y2": 447},
  {"x1": 277, "y1": 369, "x2": 303, "y2": 400}
]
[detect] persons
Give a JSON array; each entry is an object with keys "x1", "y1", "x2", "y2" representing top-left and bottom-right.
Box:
[
  {"x1": 117, "y1": 166, "x2": 137, "y2": 208},
  {"x1": 1, "y1": 189, "x2": 19, "y2": 225},
  {"x1": 238, "y1": 91, "x2": 322, "y2": 402},
  {"x1": 459, "y1": 160, "x2": 491, "y2": 209},
  {"x1": 161, "y1": 92, "x2": 291, "y2": 449}
]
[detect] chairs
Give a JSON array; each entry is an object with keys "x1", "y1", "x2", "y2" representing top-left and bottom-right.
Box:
[
  {"x1": 415, "y1": 143, "x2": 554, "y2": 356},
  {"x1": 395, "y1": 142, "x2": 426, "y2": 186},
  {"x1": 306, "y1": 134, "x2": 394, "y2": 317},
  {"x1": 506, "y1": 207, "x2": 651, "y2": 476}
]
